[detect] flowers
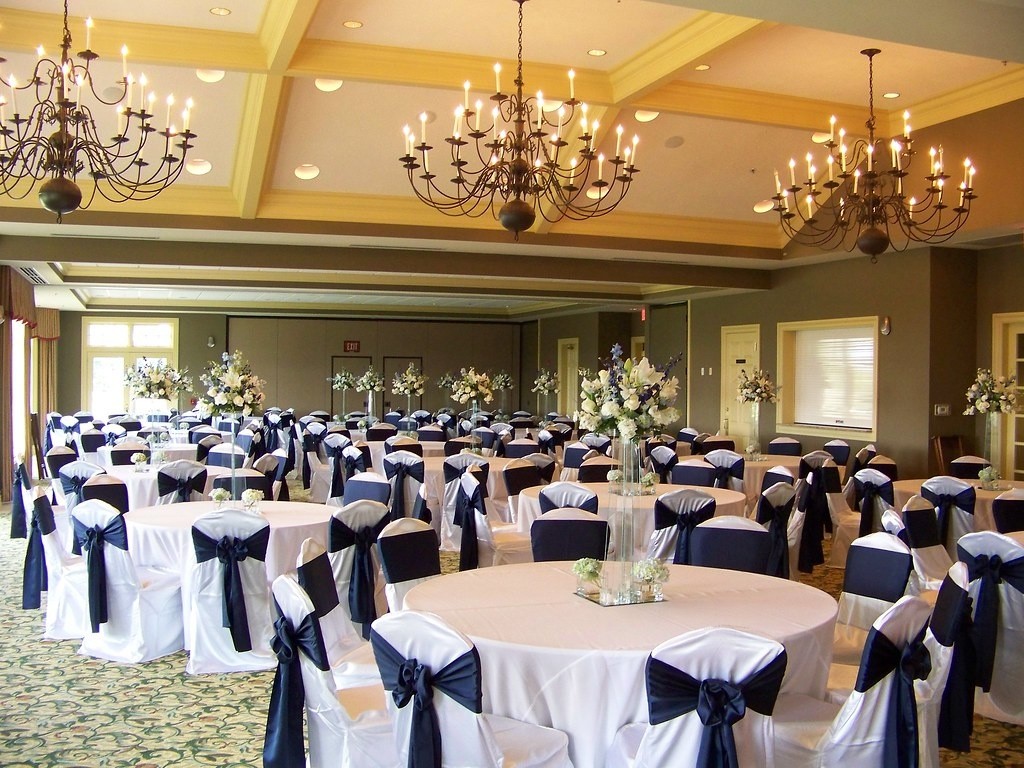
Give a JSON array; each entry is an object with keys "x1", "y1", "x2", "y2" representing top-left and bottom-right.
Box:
[{"x1": 123, "y1": 343, "x2": 1024, "y2": 585}]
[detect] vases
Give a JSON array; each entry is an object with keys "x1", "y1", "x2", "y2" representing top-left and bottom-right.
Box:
[
  {"x1": 573, "y1": 402, "x2": 1015, "y2": 606},
  {"x1": 138, "y1": 390, "x2": 551, "y2": 511}
]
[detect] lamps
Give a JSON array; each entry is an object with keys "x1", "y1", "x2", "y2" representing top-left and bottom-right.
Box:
[
  {"x1": 0, "y1": 0, "x2": 198, "y2": 224},
  {"x1": 399, "y1": 0, "x2": 640, "y2": 241},
  {"x1": 772, "y1": 48, "x2": 978, "y2": 264}
]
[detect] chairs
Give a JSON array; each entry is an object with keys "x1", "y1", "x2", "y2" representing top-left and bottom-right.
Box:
[{"x1": 11, "y1": 409, "x2": 1024, "y2": 768}]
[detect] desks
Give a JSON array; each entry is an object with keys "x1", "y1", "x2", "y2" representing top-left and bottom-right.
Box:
[
  {"x1": 677, "y1": 455, "x2": 805, "y2": 519},
  {"x1": 1003, "y1": 531, "x2": 1024, "y2": 547},
  {"x1": 123, "y1": 500, "x2": 343, "y2": 581},
  {"x1": 516, "y1": 483, "x2": 746, "y2": 561},
  {"x1": 402, "y1": 559, "x2": 839, "y2": 768},
  {"x1": 892, "y1": 479, "x2": 1024, "y2": 533},
  {"x1": 92, "y1": 422, "x2": 690, "y2": 510}
]
